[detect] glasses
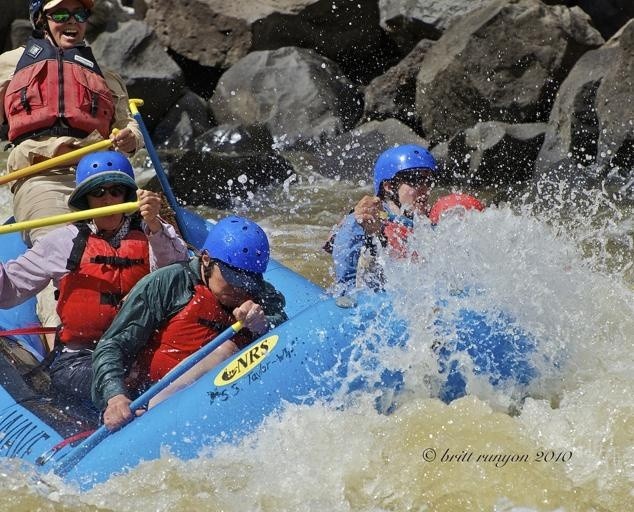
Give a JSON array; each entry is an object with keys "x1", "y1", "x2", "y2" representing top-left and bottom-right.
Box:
[
  {"x1": 50, "y1": 6, "x2": 92, "y2": 25},
  {"x1": 217, "y1": 263, "x2": 260, "y2": 292},
  {"x1": 87, "y1": 181, "x2": 127, "y2": 198},
  {"x1": 396, "y1": 172, "x2": 437, "y2": 191}
]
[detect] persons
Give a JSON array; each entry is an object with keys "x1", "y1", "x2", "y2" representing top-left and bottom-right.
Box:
[
  {"x1": 0, "y1": 148, "x2": 191, "y2": 413},
  {"x1": 0, "y1": 0, "x2": 148, "y2": 353},
  {"x1": 328, "y1": 143, "x2": 441, "y2": 295},
  {"x1": 89, "y1": 212, "x2": 288, "y2": 433}
]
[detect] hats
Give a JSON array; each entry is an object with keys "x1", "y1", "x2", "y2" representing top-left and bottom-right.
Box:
[{"x1": 41, "y1": 0, "x2": 95, "y2": 12}]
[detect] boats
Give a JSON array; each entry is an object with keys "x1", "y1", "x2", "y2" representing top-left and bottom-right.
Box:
[{"x1": 0, "y1": 188, "x2": 561, "y2": 494}]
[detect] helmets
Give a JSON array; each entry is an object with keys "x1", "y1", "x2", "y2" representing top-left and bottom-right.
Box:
[
  {"x1": 429, "y1": 191, "x2": 485, "y2": 225},
  {"x1": 201, "y1": 215, "x2": 270, "y2": 274},
  {"x1": 69, "y1": 151, "x2": 137, "y2": 206},
  {"x1": 374, "y1": 144, "x2": 439, "y2": 196}
]
